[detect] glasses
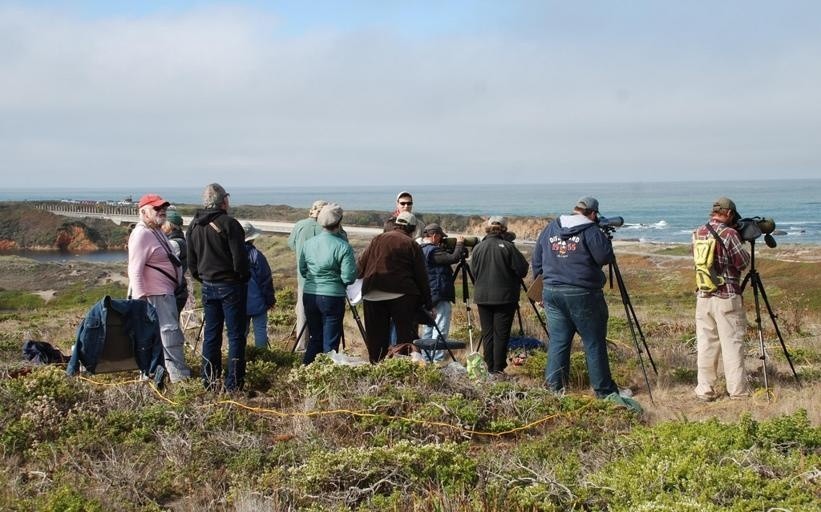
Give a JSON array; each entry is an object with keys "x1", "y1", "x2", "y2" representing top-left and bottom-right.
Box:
[{"x1": 153, "y1": 204, "x2": 167, "y2": 211}]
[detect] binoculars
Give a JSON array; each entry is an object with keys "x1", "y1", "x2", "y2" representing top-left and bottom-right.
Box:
[
  {"x1": 597, "y1": 216, "x2": 624, "y2": 228},
  {"x1": 441, "y1": 237, "x2": 478, "y2": 248},
  {"x1": 737, "y1": 218, "x2": 777, "y2": 247}
]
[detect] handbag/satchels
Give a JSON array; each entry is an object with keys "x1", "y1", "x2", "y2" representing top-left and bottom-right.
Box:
[
  {"x1": 174, "y1": 275, "x2": 189, "y2": 313},
  {"x1": 23, "y1": 340, "x2": 70, "y2": 364}
]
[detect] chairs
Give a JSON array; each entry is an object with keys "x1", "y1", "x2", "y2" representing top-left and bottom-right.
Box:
[{"x1": 412, "y1": 308, "x2": 465, "y2": 363}]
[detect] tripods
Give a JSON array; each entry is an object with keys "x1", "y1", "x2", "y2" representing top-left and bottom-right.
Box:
[
  {"x1": 605, "y1": 232, "x2": 658, "y2": 405},
  {"x1": 291, "y1": 295, "x2": 369, "y2": 354},
  {"x1": 451, "y1": 261, "x2": 550, "y2": 358},
  {"x1": 740, "y1": 241, "x2": 803, "y2": 405}
]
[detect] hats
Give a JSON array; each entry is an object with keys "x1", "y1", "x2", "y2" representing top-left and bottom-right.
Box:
[
  {"x1": 397, "y1": 191, "x2": 413, "y2": 202},
  {"x1": 424, "y1": 223, "x2": 448, "y2": 237},
  {"x1": 396, "y1": 211, "x2": 418, "y2": 226},
  {"x1": 309, "y1": 201, "x2": 343, "y2": 227},
  {"x1": 713, "y1": 198, "x2": 741, "y2": 219},
  {"x1": 577, "y1": 197, "x2": 601, "y2": 214},
  {"x1": 239, "y1": 222, "x2": 262, "y2": 242},
  {"x1": 400, "y1": 202, "x2": 412, "y2": 205},
  {"x1": 488, "y1": 216, "x2": 508, "y2": 229},
  {"x1": 165, "y1": 210, "x2": 181, "y2": 225},
  {"x1": 203, "y1": 183, "x2": 230, "y2": 206},
  {"x1": 139, "y1": 195, "x2": 170, "y2": 208}
]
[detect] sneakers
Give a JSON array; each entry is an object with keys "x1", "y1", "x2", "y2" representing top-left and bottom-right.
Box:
[{"x1": 617, "y1": 387, "x2": 634, "y2": 399}]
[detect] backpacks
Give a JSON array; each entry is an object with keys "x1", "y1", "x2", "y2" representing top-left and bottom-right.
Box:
[{"x1": 693, "y1": 226, "x2": 728, "y2": 294}]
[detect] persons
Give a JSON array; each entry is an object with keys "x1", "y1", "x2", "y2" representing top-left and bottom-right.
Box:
[
  {"x1": 126, "y1": 183, "x2": 277, "y2": 393},
  {"x1": 531, "y1": 196, "x2": 619, "y2": 402},
  {"x1": 691, "y1": 197, "x2": 751, "y2": 402},
  {"x1": 355, "y1": 191, "x2": 466, "y2": 365},
  {"x1": 287, "y1": 200, "x2": 357, "y2": 368},
  {"x1": 471, "y1": 216, "x2": 528, "y2": 376}
]
